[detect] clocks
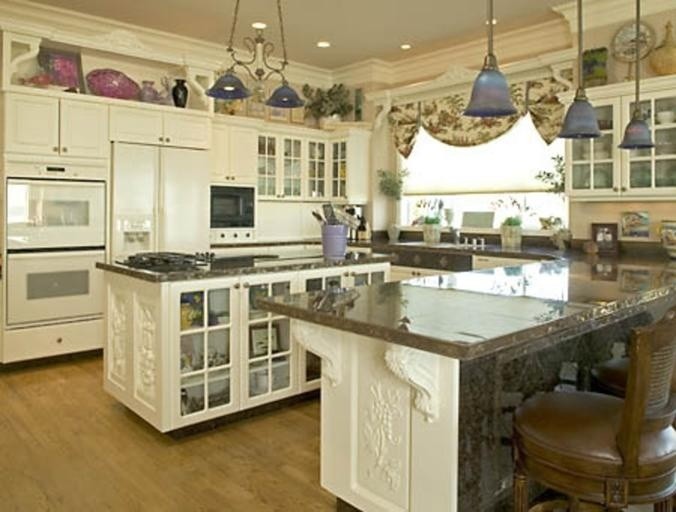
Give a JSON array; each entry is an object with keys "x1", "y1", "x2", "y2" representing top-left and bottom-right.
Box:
[{"x1": 610, "y1": 19, "x2": 654, "y2": 64}]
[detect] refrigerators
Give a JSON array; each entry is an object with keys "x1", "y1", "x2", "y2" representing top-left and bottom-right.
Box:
[{"x1": 107, "y1": 139, "x2": 212, "y2": 262}]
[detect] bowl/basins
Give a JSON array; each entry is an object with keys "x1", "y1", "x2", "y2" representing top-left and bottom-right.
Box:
[
  {"x1": 598, "y1": 119, "x2": 612, "y2": 131},
  {"x1": 582, "y1": 142, "x2": 604, "y2": 152},
  {"x1": 656, "y1": 110, "x2": 674, "y2": 126},
  {"x1": 583, "y1": 169, "x2": 608, "y2": 187}
]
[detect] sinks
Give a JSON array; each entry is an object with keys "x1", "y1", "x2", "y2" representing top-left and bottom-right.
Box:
[{"x1": 463, "y1": 245, "x2": 494, "y2": 250}]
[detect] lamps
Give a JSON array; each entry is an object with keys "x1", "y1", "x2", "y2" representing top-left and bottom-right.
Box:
[
  {"x1": 460, "y1": 0, "x2": 516, "y2": 117},
  {"x1": 205, "y1": 1, "x2": 305, "y2": 109},
  {"x1": 619, "y1": 1, "x2": 655, "y2": 149},
  {"x1": 560, "y1": 0, "x2": 600, "y2": 139}
]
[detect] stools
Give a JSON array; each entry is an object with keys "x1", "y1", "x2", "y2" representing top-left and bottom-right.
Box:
[{"x1": 587, "y1": 356, "x2": 676, "y2": 403}]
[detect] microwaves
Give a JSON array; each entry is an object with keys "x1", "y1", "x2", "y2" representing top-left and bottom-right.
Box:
[{"x1": 210, "y1": 185, "x2": 254, "y2": 228}]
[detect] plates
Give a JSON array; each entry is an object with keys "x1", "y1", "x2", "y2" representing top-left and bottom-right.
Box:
[{"x1": 581, "y1": 152, "x2": 609, "y2": 159}]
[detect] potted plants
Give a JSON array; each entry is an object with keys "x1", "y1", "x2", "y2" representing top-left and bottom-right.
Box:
[
  {"x1": 423, "y1": 217, "x2": 440, "y2": 241},
  {"x1": 300, "y1": 81, "x2": 351, "y2": 128},
  {"x1": 501, "y1": 216, "x2": 522, "y2": 247},
  {"x1": 377, "y1": 167, "x2": 408, "y2": 241}
]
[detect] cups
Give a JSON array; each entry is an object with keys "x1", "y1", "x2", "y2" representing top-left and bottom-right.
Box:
[
  {"x1": 350, "y1": 228, "x2": 356, "y2": 240},
  {"x1": 255, "y1": 370, "x2": 276, "y2": 390}
]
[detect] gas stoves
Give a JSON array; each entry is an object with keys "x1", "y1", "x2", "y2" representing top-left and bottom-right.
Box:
[{"x1": 118, "y1": 249, "x2": 215, "y2": 273}]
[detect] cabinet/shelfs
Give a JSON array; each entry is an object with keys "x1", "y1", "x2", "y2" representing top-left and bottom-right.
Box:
[
  {"x1": 0, "y1": 84, "x2": 110, "y2": 160},
  {"x1": 552, "y1": 73, "x2": 676, "y2": 203},
  {"x1": 298, "y1": 262, "x2": 390, "y2": 398},
  {"x1": 326, "y1": 128, "x2": 372, "y2": 206},
  {"x1": 111, "y1": 97, "x2": 212, "y2": 151},
  {"x1": 206, "y1": 113, "x2": 264, "y2": 185},
  {"x1": 303, "y1": 127, "x2": 329, "y2": 201},
  {"x1": 256, "y1": 123, "x2": 304, "y2": 201},
  {"x1": 101, "y1": 268, "x2": 299, "y2": 435}
]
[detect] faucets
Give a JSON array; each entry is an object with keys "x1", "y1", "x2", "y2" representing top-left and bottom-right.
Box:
[
  {"x1": 471, "y1": 237, "x2": 477, "y2": 245},
  {"x1": 448, "y1": 226, "x2": 462, "y2": 245}
]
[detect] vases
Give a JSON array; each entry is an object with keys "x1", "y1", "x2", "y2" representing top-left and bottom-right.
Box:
[
  {"x1": 171, "y1": 79, "x2": 189, "y2": 108},
  {"x1": 138, "y1": 80, "x2": 158, "y2": 103}
]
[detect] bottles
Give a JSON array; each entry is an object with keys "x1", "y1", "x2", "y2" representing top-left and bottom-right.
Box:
[
  {"x1": 356, "y1": 220, "x2": 367, "y2": 240},
  {"x1": 181, "y1": 298, "x2": 196, "y2": 329},
  {"x1": 180, "y1": 390, "x2": 193, "y2": 416}
]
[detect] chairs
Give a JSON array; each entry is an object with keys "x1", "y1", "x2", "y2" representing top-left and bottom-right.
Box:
[{"x1": 509, "y1": 305, "x2": 676, "y2": 511}]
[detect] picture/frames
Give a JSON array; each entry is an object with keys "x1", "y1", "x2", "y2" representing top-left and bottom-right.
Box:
[{"x1": 590, "y1": 223, "x2": 619, "y2": 257}]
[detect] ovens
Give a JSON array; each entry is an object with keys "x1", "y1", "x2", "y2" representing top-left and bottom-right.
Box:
[{"x1": 3, "y1": 153, "x2": 112, "y2": 368}]
